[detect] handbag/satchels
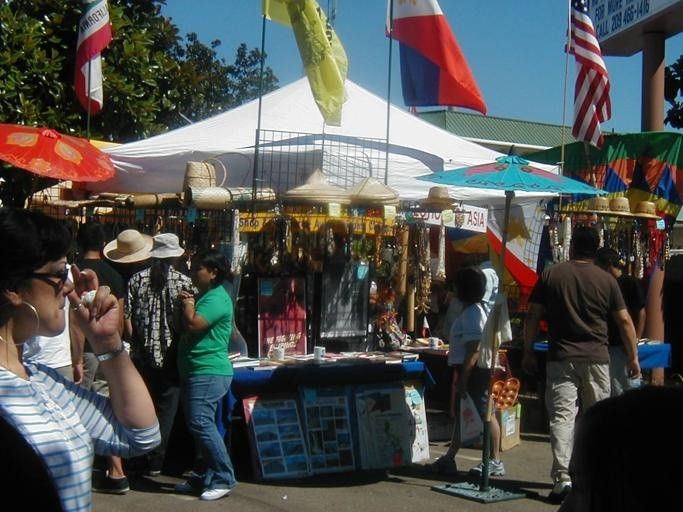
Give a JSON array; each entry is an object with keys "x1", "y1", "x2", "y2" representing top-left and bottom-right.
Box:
[{"x1": 184, "y1": 159, "x2": 227, "y2": 190}]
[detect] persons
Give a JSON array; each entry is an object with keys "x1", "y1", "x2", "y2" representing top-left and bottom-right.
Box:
[
  {"x1": 522, "y1": 226, "x2": 641, "y2": 505},
  {"x1": 423, "y1": 269, "x2": 507, "y2": 478},
  {"x1": 592, "y1": 246, "x2": 647, "y2": 398},
  {"x1": 123, "y1": 233, "x2": 200, "y2": 477},
  {"x1": 172, "y1": 249, "x2": 238, "y2": 502},
  {"x1": 69, "y1": 224, "x2": 132, "y2": 496},
  {"x1": 557, "y1": 387, "x2": 682, "y2": 511},
  {"x1": 102, "y1": 229, "x2": 155, "y2": 279},
  {"x1": 1, "y1": 208, "x2": 163, "y2": 512}
]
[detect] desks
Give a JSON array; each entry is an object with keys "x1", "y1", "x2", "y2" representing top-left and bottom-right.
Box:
[{"x1": 397, "y1": 344, "x2": 522, "y2": 448}]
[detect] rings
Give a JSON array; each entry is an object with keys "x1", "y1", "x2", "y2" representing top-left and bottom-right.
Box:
[{"x1": 71, "y1": 303, "x2": 82, "y2": 312}]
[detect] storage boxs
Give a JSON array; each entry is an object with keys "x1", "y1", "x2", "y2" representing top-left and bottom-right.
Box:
[{"x1": 501, "y1": 405, "x2": 521, "y2": 451}]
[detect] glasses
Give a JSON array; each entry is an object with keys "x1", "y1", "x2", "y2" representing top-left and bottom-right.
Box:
[{"x1": 4, "y1": 267, "x2": 72, "y2": 284}]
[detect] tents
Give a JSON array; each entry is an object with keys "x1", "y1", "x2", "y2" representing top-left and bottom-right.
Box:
[{"x1": 79, "y1": 70, "x2": 560, "y2": 212}]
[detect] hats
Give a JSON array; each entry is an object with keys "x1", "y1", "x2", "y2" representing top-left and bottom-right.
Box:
[
  {"x1": 634, "y1": 200, "x2": 662, "y2": 219},
  {"x1": 147, "y1": 233, "x2": 185, "y2": 258},
  {"x1": 103, "y1": 229, "x2": 157, "y2": 263},
  {"x1": 419, "y1": 186, "x2": 458, "y2": 208},
  {"x1": 585, "y1": 196, "x2": 611, "y2": 216},
  {"x1": 78, "y1": 222, "x2": 107, "y2": 243},
  {"x1": 608, "y1": 198, "x2": 635, "y2": 220},
  {"x1": 282, "y1": 168, "x2": 349, "y2": 201},
  {"x1": 454, "y1": 236, "x2": 491, "y2": 255},
  {"x1": 352, "y1": 177, "x2": 399, "y2": 208}
]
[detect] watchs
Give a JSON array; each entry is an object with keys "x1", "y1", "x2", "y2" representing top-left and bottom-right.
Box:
[{"x1": 93, "y1": 341, "x2": 127, "y2": 363}]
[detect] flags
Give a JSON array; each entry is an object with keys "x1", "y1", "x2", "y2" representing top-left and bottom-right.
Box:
[
  {"x1": 385, "y1": 0, "x2": 487, "y2": 119},
  {"x1": 261, "y1": 0, "x2": 350, "y2": 127},
  {"x1": 563, "y1": 0, "x2": 612, "y2": 151},
  {"x1": 74, "y1": 1, "x2": 111, "y2": 115}
]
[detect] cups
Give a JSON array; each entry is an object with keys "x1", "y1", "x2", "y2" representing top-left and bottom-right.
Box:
[
  {"x1": 430, "y1": 338, "x2": 443, "y2": 351},
  {"x1": 267, "y1": 348, "x2": 284, "y2": 361},
  {"x1": 314, "y1": 347, "x2": 325, "y2": 360}
]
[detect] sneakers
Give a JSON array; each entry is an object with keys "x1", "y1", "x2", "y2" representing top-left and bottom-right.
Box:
[
  {"x1": 175, "y1": 481, "x2": 196, "y2": 492},
  {"x1": 549, "y1": 478, "x2": 572, "y2": 500},
  {"x1": 92, "y1": 471, "x2": 129, "y2": 493},
  {"x1": 425, "y1": 456, "x2": 457, "y2": 474},
  {"x1": 201, "y1": 488, "x2": 231, "y2": 500},
  {"x1": 470, "y1": 460, "x2": 504, "y2": 476}
]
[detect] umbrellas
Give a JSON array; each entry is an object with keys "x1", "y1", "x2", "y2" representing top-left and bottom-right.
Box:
[
  {"x1": 412, "y1": 142, "x2": 610, "y2": 283},
  {"x1": 0, "y1": 122, "x2": 117, "y2": 211}
]
